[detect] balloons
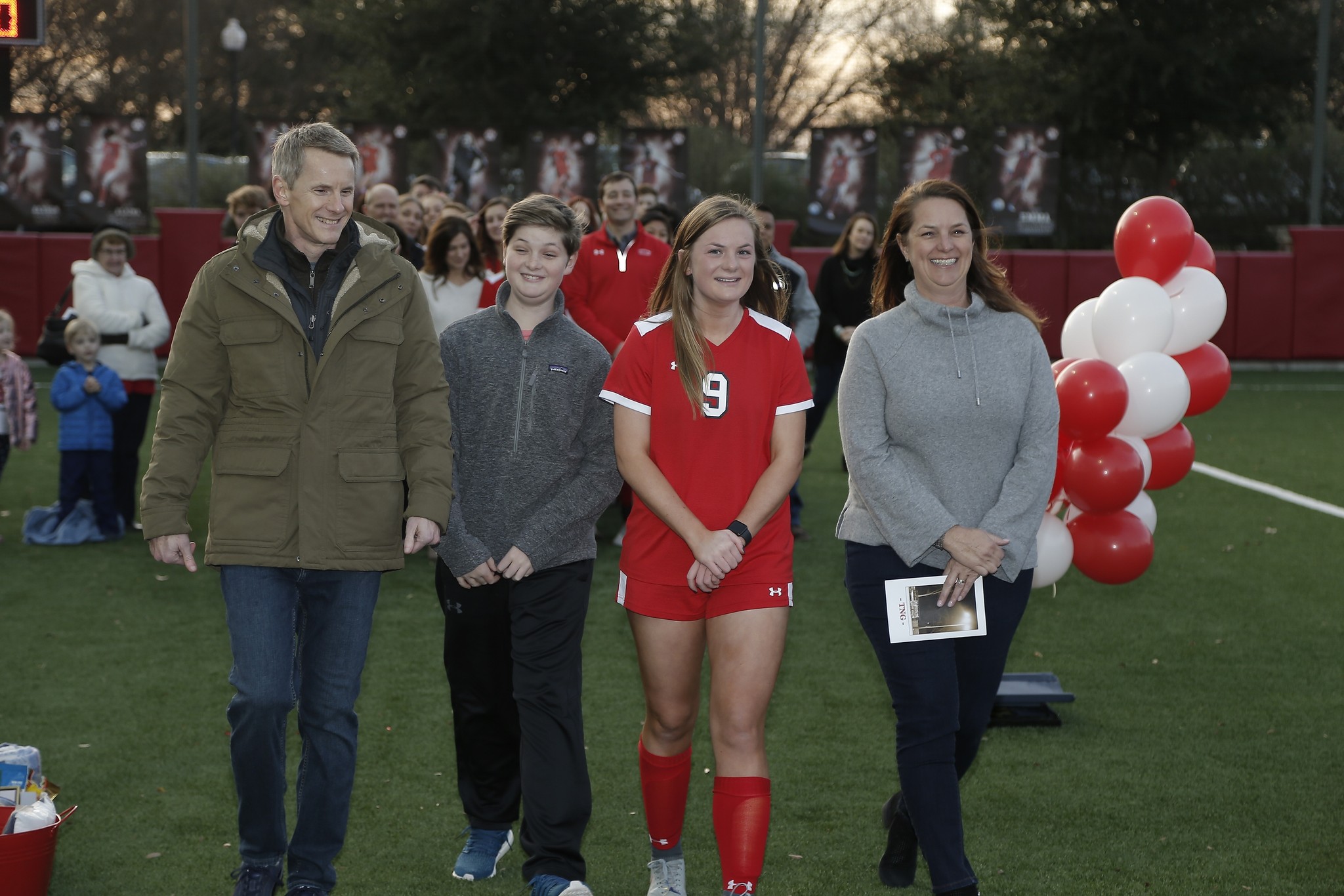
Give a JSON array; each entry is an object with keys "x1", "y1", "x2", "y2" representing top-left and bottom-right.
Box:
[
  {"x1": 1114, "y1": 195, "x2": 1194, "y2": 286},
  {"x1": 1111, "y1": 352, "x2": 1191, "y2": 440},
  {"x1": 1064, "y1": 434, "x2": 1144, "y2": 515},
  {"x1": 1030, "y1": 231, "x2": 1232, "y2": 589}
]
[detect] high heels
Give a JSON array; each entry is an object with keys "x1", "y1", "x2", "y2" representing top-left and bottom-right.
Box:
[{"x1": 878, "y1": 792, "x2": 918, "y2": 889}]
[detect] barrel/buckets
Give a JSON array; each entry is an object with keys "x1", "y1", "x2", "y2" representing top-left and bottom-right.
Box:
[{"x1": 0, "y1": 804, "x2": 78, "y2": 896}]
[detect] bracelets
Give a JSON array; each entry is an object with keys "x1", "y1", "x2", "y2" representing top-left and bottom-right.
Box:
[{"x1": 934, "y1": 533, "x2": 945, "y2": 551}]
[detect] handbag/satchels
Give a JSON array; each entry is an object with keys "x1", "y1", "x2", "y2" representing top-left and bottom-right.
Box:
[{"x1": 37, "y1": 314, "x2": 76, "y2": 366}]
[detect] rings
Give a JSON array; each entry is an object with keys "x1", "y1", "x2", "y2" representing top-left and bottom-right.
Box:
[{"x1": 957, "y1": 578, "x2": 966, "y2": 585}]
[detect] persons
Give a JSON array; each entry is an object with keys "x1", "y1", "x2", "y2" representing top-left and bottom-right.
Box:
[
  {"x1": 790, "y1": 212, "x2": 882, "y2": 471},
  {"x1": 635, "y1": 185, "x2": 681, "y2": 245},
  {"x1": 437, "y1": 193, "x2": 623, "y2": 896},
  {"x1": 0, "y1": 310, "x2": 40, "y2": 516},
  {"x1": 139, "y1": 124, "x2": 454, "y2": 896},
  {"x1": 595, "y1": 195, "x2": 813, "y2": 896},
  {"x1": 748, "y1": 203, "x2": 822, "y2": 540},
  {"x1": 50, "y1": 317, "x2": 128, "y2": 543},
  {"x1": 216, "y1": 184, "x2": 275, "y2": 254},
  {"x1": 836, "y1": 178, "x2": 1061, "y2": 896},
  {"x1": 72, "y1": 229, "x2": 172, "y2": 528},
  {"x1": 358, "y1": 173, "x2": 601, "y2": 338},
  {"x1": 562, "y1": 171, "x2": 672, "y2": 548}
]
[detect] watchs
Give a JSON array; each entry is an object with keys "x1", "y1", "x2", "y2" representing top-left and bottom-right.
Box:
[{"x1": 727, "y1": 520, "x2": 752, "y2": 547}]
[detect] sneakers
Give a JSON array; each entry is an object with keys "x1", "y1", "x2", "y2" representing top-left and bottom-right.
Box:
[
  {"x1": 230, "y1": 858, "x2": 284, "y2": 895},
  {"x1": 520, "y1": 874, "x2": 592, "y2": 896},
  {"x1": 646, "y1": 859, "x2": 687, "y2": 896},
  {"x1": 452, "y1": 827, "x2": 514, "y2": 881},
  {"x1": 285, "y1": 886, "x2": 328, "y2": 896}
]
